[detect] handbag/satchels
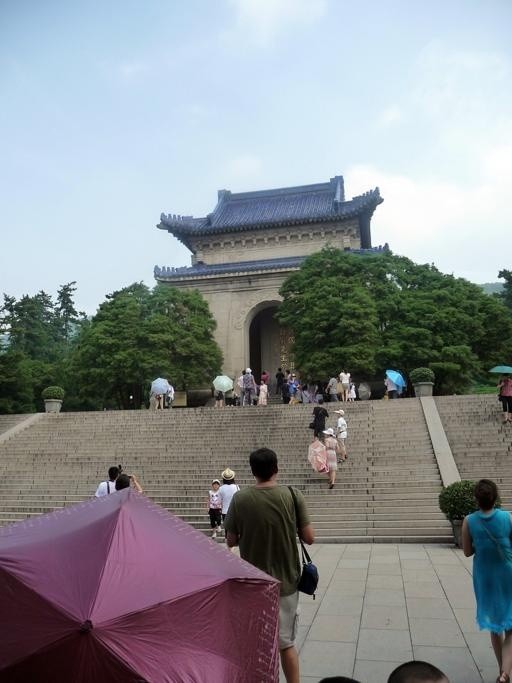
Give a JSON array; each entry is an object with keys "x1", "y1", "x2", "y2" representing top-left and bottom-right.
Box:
[
  {"x1": 297, "y1": 560, "x2": 320, "y2": 597},
  {"x1": 336, "y1": 383, "x2": 343, "y2": 394},
  {"x1": 309, "y1": 422, "x2": 315, "y2": 429},
  {"x1": 253, "y1": 394, "x2": 259, "y2": 401}
]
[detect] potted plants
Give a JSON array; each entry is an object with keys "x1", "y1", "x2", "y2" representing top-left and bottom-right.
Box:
[
  {"x1": 408, "y1": 367, "x2": 436, "y2": 394},
  {"x1": 41, "y1": 385, "x2": 68, "y2": 412},
  {"x1": 439, "y1": 478, "x2": 503, "y2": 545}
]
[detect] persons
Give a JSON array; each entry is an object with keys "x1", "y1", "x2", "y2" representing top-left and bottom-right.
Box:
[
  {"x1": 223, "y1": 446, "x2": 315, "y2": 682},
  {"x1": 115, "y1": 473, "x2": 143, "y2": 492},
  {"x1": 312, "y1": 398, "x2": 329, "y2": 440},
  {"x1": 460, "y1": 478, "x2": 512, "y2": 682},
  {"x1": 333, "y1": 408, "x2": 348, "y2": 463},
  {"x1": 218, "y1": 466, "x2": 241, "y2": 538},
  {"x1": 322, "y1": 427, "x2": 340, "y2": 489},
  {"x1": 496, "y1": 381, "x2": 512, "y2": 423},
  {"x1": 208, "y1": 478, "x2": 222, "y2": 537},
  {"x1": 93, "y1": 466, "x2": 120, "y2": 498},
  {"x1": 149, "y1": 366, "x2": 409, "y2": 410},
  {"x1": 387, "y1": 659, "x2": 452, "y2": 682}
]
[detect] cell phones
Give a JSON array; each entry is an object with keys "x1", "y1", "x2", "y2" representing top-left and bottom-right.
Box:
[{"x1": 127, "y1": 475, "x2": 132, "y2": 479}]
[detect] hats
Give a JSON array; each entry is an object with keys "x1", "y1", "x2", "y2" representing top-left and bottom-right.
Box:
[
  {"x1": 334, "y1": 410, "x2": 345, "y2": 416},
  {"x1": 322, "y1": 428, "x2": 334, "y2": 436},
  {"x1": 291, "y1": 373, "x2": 297, "y2": 377},
  {"x1": 210, "y1": 467, "x2": 236, "y2": 485},
  {"x1": 245, "y1": 367, "x2": 253, "y2": 374}
]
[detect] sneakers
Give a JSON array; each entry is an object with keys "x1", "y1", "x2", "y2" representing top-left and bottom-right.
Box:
[
  {"x1": 327, "y1": 454, "x2": 348, "y2": 490},
  {"x1": 212, "y1": 525, "x2": 223, "y2": 540}
]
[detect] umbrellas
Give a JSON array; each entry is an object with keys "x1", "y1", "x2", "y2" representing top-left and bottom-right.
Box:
[
  {"x1": 1, "y1": 487, "x2": 285, "y2": 682},
  {"x1": 307, "y1": 438, "x2": 327, "y2": 471},
  {"x1": 487, "y1": 364, "x2": 512, "y2": 382}
]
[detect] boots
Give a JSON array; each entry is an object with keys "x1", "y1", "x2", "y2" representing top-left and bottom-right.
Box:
[{"x1": 502, "y1": 412, "x2": 512, "y2": 424}]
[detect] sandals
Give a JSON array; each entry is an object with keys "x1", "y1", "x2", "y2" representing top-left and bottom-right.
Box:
[{"x1": 496, "y1": 671, "x2": 511, "y2": 682}]
